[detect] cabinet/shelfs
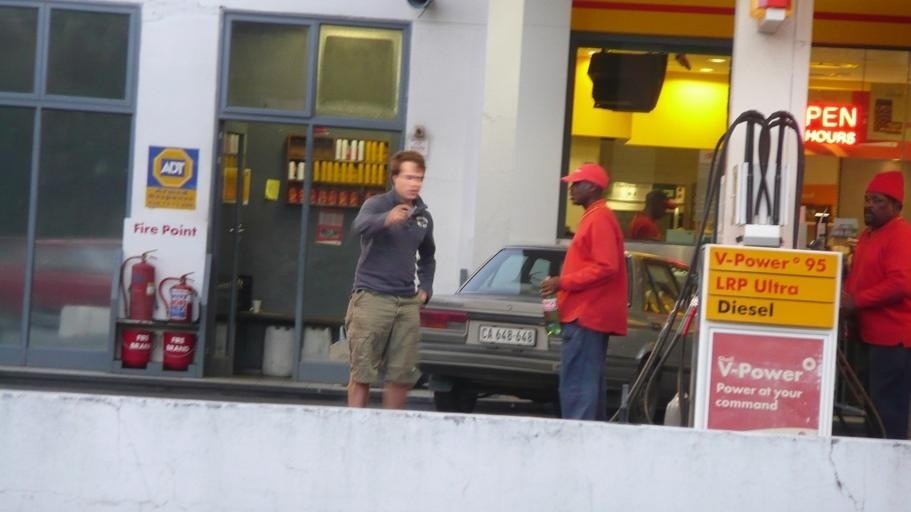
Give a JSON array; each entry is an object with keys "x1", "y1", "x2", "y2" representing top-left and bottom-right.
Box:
[{"x1": 285, "y1": 133, "x2": 390, "y2": 210}]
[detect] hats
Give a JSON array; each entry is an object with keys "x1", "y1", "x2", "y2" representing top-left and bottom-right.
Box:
[
  {"x1": 645, "y1": 190, "x2": 679, "y2": 210},
  {"x1": 561, "y1": 162, "x2": 610, "y2": 192},
  {"x1": 865, "y1": 170, "x2": 904, "y2": 210}
]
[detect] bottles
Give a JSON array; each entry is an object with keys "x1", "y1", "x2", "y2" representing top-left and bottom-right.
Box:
[
  {"x1": 287, "y1": 138, "x2": 388, "y2": 206},
  {"x1": 542, "y1": 276, "x2": 562, "y2": 337}
]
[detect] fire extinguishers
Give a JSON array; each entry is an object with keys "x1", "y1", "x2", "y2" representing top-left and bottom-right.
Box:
[{"x1": 121, "y1": 248, "x2": 202, "y2": 323}]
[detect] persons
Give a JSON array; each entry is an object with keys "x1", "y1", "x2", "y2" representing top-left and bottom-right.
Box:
[
  {"x1": 344, "y1": 150, "x2": 435, "y2": 409},
  {"x1": 839, "y1": 170, "x2": 911, "y2": 438},
  {"x1": 540, "y1": 161, "x2": 628, "y2": 420},
  {"x1": 625, "y1": 190, "x2": 677, "y2": 240}
]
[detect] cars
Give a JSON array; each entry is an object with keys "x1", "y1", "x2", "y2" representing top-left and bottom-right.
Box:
[
  {"x1": 0, "y1": 235, "x2": 125, "y2": 317},
  {"x1": 417, "y1": 238, "x2": 697, "y2": 428}
]
